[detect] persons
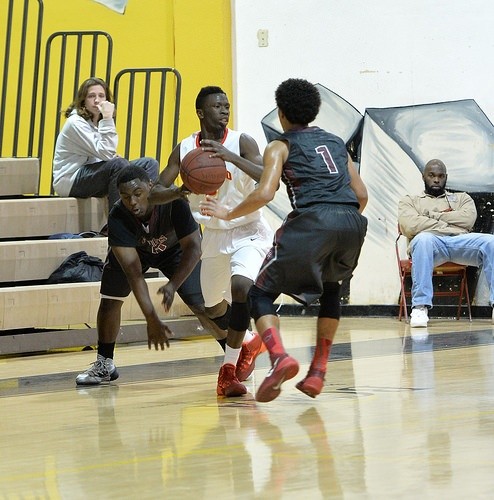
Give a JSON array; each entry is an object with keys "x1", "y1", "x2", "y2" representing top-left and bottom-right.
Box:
[
  {"x1": 397, "y1": 158, "x2": 494, "y2": 328},
  {"x1": 147, "y1": 86, "x2": 274, "y2": 395},
  {"x1": 199, "y1": 78, "x2": 368, "y2": 403},
  {"x1": 53, "y1": 76, "x2": 159, "y2": 237},
  {"x1": 76, "y1": 164, "x2": 227, "y2": 386}
]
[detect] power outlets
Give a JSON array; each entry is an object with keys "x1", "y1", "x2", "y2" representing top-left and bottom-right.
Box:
[{"x1": 258, "y1": 29, "x2": 268, "y2": 47}]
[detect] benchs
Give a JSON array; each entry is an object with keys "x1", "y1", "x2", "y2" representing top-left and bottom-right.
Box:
[{"x1": 0, "y1": 156, "x2": 196, "y2": 333}]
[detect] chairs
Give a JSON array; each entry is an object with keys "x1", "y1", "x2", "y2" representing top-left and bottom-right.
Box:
[{"x1": 395, "y1": 231, "x2": 472, "y2": 322}]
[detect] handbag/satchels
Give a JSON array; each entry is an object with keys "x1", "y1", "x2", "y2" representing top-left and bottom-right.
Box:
[{"x1": 47, "y1": 251, "x2": 103, "y2": 282}]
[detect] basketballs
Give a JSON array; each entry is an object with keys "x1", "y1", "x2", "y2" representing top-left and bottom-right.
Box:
[{"x1": 180, "y1": 148, "x2": 226, "y2": 194}]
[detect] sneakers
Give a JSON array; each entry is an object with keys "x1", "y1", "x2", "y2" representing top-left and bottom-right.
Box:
[
  {"x1": 217, "y1": 363, "x2": 247, "y2": 396},
  {"x1": 410, "y1": 308, "x2": 429, "y2": 327},
  {"x1": 254, "y1": 354, "x2": 299, "y2": 402},
  {"x1": 296, "y1": 369, "x2": 324, "y2": 399},
  {"x1": 234, "y1": 332, "x2": 268, "y2": 381},
  {"x1": 74, "y1": 354, "x2": 120, "y2": 385}
]
